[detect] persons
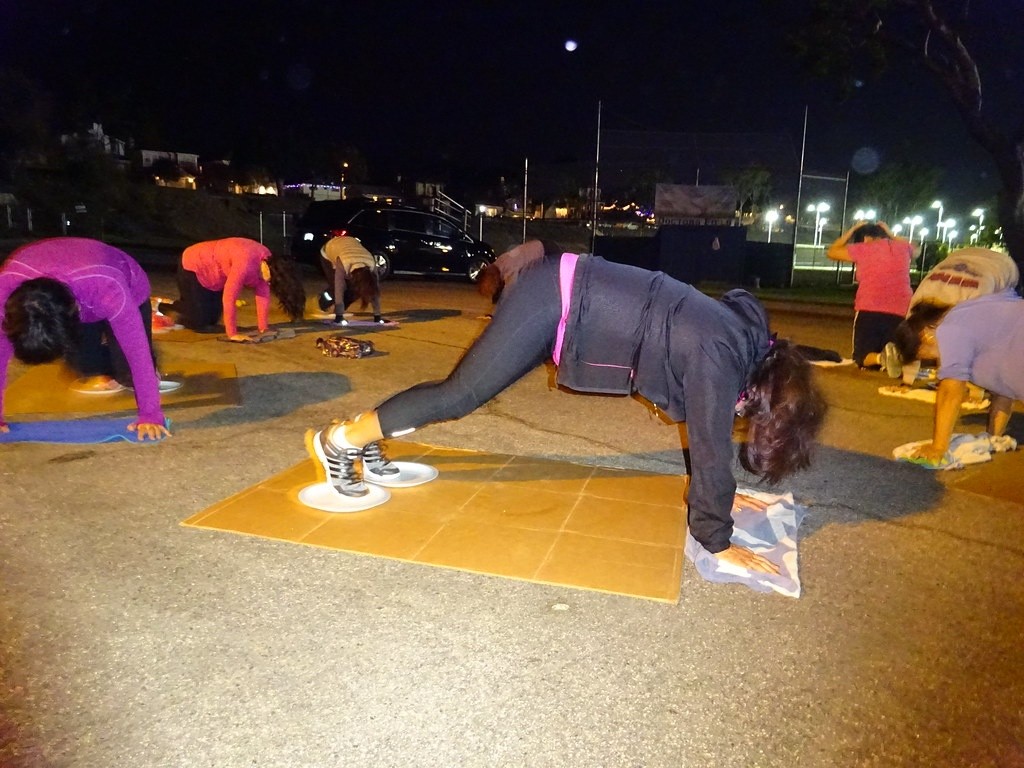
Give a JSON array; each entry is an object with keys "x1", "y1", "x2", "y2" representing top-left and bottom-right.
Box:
[
  {"x1": 826, "y1": 220, "x2": 914, "y2": 378},
  {"x1": 318, "y1": 236, "x2": 399, "y2": 327},
  {"x1": 476, "y1": 240, "x2": 562, "y2": 319},
  {"x1": 896, "y1": 289, "x2": 1024, "y2": 464},
  {"x1": 891, "y1": 247, "x2": 1021, "y2": 393},
  {"x1": 0, "y1": 239, "x2": 173, "y2": 441},
  {"x1": 150, "y1": 236, "x2": 307, "y2": 341},
  {"x1": 312, "y1": 252, "x2": 828, "y2": 576}
]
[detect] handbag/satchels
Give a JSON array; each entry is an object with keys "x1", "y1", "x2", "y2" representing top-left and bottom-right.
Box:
[{"x1": 315, "y1": 334, "x2": 375, "y2": 359}]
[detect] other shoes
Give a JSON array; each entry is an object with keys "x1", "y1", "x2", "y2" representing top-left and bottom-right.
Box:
[
  {"x1": 311, "y1": 296, "x2": 325, "y2": 314},
  {"x1": 150, "y1": 296, "x2": 175, "y2": 329}
]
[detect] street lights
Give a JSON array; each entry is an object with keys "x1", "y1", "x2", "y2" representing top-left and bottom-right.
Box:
[
  {"x1": 903, "y1": 216, "x2": 923, "y2": 243},
  {"x1": 807, "y1": 201, "x2": 830, "y2": 245},
  {"x1": 765, "y1": 210, "x2": 779, "y2": 243},
  {"x1": 931, "y1": 199, "x2": 943, "y2": 239}
]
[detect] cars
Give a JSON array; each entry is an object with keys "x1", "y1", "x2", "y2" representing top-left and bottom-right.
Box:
[{"x1": 290, "y1": 200, "x2": 497, "y2": 284}]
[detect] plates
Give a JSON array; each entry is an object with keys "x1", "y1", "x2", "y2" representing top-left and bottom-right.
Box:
[
  {"x1": 128, "y1": 380, "x2": 183, "y2": 392},
  {"x1": 363, "y1": 460, "x2": 441, "y2": 489},
  {"x1": 297, "y1": 479, "x2": 388, "y2": 513},
  {"x1": 69, "y1": 377, "x2": 125, "y2": 396}
]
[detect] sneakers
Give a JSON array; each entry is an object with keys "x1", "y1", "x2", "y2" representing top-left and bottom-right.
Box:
[
  {"x1": 312, "y1": 424, "x2": 370, "y2": 497},
  {"x1": 351, "y1": 413, "x2": 401, "y2": 481},
  {"x1": 880, "y1": 343, "x2": 901, "y2": 379}
]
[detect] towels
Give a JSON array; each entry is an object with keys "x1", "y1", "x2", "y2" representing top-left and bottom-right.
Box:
[
  {"x1": 0, "y1": 416, "x2": 173, "y2": 444},
  {"x1": 686, "y1": 488, "x2": 811, "y2": 599},
  {"x1": 216, "y1": 328, "x2": 296, "y2": 343},
  {"x1": 879, "y1": 386, "x2": 991, "y2": 410},
  {"x1": 892, "y1": 433, "x2": 1018, "y2": 471},
  {"x1": 322, "y1": 320, "x2": 399, "y2": 325}
]
